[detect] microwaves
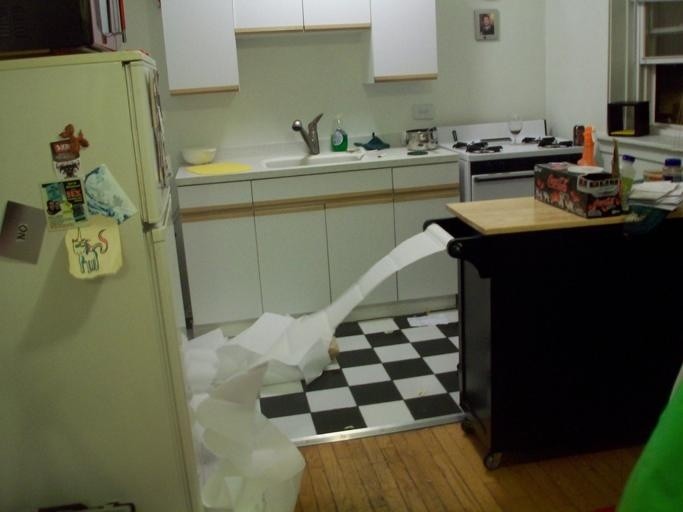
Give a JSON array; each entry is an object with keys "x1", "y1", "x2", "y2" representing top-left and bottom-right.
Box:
[{"x1": 0, "y1": 0, "x2": 128, "y2": 59}]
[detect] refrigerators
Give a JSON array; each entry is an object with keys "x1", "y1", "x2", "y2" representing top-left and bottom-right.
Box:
[{"x1": 0, "y1": 50, "x2": 202, "y2": 512}]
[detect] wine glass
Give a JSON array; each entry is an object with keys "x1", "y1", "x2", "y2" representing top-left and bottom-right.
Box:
[{"x1": 508, "y1": 111, "x2": 523, "y2": 145}]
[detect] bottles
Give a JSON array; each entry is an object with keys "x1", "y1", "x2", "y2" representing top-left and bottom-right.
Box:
[
  {"x1": 662, "y1": 158, "x2": 683, "y2": 183},
  {"x1": 618, "y1": 153, "x2": 637, "y2": 211},
  {"x1": 573, "y1": 119, "x2": 586, "y2": 146}
]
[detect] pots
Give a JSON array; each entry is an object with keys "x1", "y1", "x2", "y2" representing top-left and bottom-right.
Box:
[{"x1": 405, "y1": 126, "x2": 439, "y2": 152}]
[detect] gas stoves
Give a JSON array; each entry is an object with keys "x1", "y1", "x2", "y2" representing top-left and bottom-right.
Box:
[{"x1": 440, "y1": 135, "x2": 584, "y2": 176}]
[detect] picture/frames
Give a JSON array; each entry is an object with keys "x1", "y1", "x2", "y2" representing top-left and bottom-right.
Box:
[{"x1": 472, "y1": 8, "x2": 500, "y2": 42}]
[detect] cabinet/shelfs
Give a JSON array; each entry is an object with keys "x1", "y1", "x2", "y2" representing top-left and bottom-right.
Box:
[
  {"x1": 233, "y1": 1, "x2": 372, "y2": 36},
  {"x1": 370, "y1": 0, "x2": 439, "y2": 83},
  {"x1": 174, "y1": 154, "x2": 464, "y2": 341},
  {"x1": 422, "y1": 191, "x2": 683, "y2": 474},
  {"x1": 160, "y1": 1, "x2": 240, "y2": 97}
]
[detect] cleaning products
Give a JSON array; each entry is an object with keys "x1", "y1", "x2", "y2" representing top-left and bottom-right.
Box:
[{"x1": 576, "y1": 125, "x2": 597, "y2": 166}]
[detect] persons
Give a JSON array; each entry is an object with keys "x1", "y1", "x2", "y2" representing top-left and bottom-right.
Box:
[{"x1": 480, "y1": 14, "x2": 493, "y2": 34}]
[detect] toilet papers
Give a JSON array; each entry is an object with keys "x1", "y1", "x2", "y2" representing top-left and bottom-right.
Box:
[{"x1": 180, "y1": 223, "x2": 456, "y2": 512}]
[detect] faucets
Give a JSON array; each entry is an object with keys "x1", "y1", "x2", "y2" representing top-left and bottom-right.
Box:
[{"x1": 291, "y1": 114, "x2": 323, "y2": 154}]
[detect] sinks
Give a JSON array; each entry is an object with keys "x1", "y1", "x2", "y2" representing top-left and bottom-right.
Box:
[{"x1": 264, "y1": 156, "x2": 362, "y2": 168}]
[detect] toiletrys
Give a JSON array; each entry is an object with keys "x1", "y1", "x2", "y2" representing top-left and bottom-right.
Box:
[{"x1": 331, "y1": 113, "x2": 349, "y2": 151}]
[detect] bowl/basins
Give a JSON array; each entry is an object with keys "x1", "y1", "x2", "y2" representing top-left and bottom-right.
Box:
[{"x1": 180, "y1": 146, "x2": 218, "y2": 165}]
